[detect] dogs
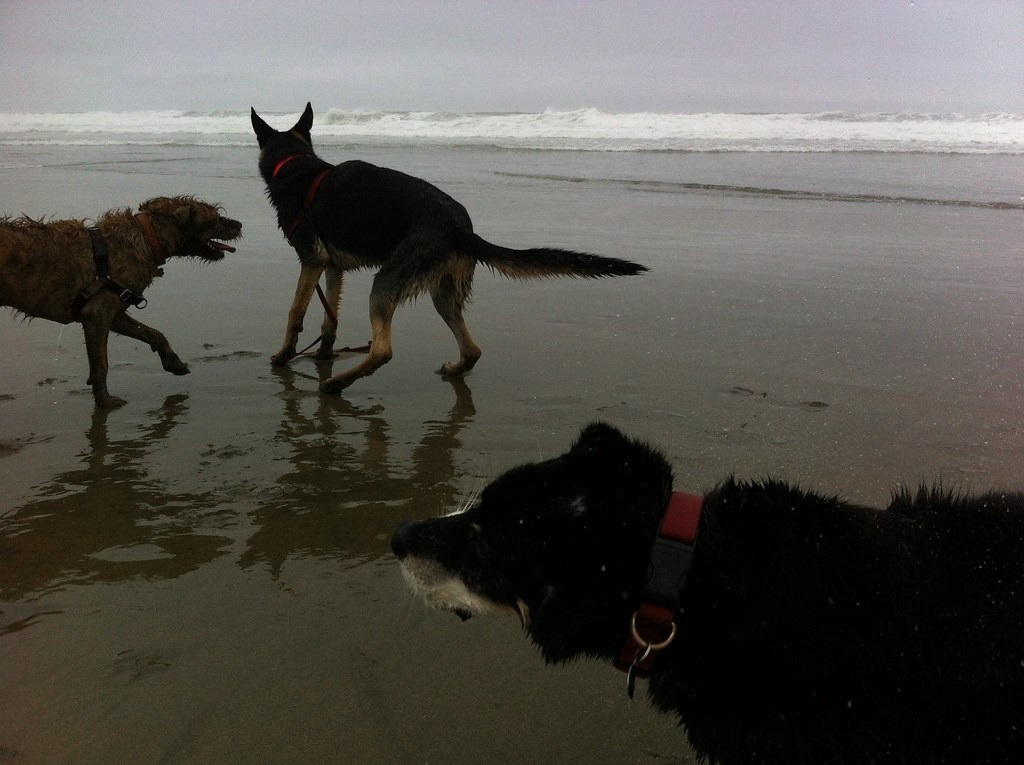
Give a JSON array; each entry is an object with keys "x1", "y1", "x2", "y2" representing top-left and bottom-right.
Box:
[
  {"x1": 391, "y1": 424, "x2": 1024, "y2": 765},
  {"x1": 0, "y1": 194, "x2": 245, "y2": 409},
  {"x1": 250, "y1": 101, "x2": 651, "y2": 395}
]
